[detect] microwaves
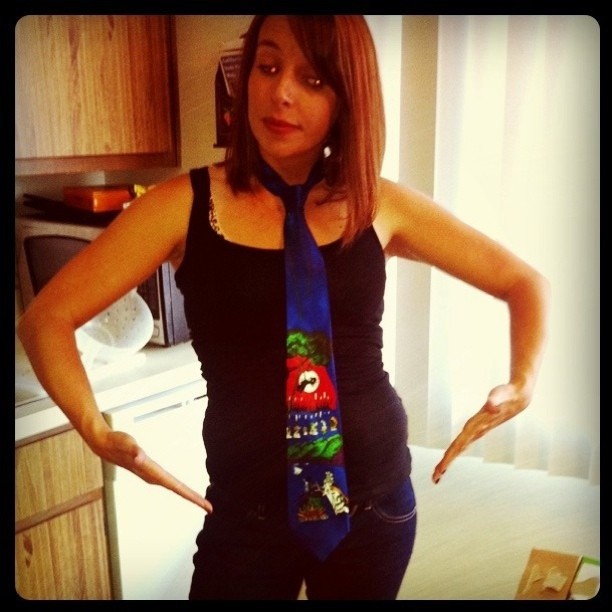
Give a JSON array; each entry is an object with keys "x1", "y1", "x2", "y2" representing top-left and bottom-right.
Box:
[{"x1": 15, "y1": 216, "x2": 190, "y2": 348}]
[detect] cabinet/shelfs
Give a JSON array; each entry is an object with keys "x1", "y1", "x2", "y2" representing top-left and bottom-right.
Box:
[
  {"x1": 16, "y1": 414, "x2": 115, "y2": 600},
  {"x1": 16, "y1": 15, "x2": 178, "y2": 177}
]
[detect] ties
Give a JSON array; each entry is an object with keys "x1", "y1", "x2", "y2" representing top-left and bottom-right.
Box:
[{"x1": 253, "y1": 153, "x2": 351, "y2": 561}]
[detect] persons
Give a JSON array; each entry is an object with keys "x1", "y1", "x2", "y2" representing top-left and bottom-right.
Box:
[{"x1": 16, "y1": 14, "x2": 550, "y2": 601}]
